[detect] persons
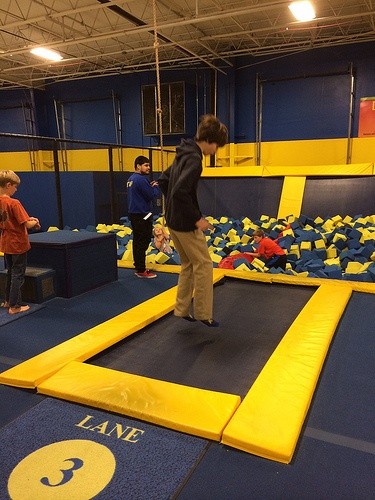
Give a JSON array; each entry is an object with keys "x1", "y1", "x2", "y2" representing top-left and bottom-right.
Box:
[
  {"x1": 126, "y1": 155, "x2": 159, "y2": 278},
  {"x1": 244, "y1": 228, "x2": 287, "y2": 272},
  {"x1": 157, "y1": 115, "x2": 229, "y2": 325},
  {"x1": 0, "y1": 169, "x2": 40, "y2": 315}
]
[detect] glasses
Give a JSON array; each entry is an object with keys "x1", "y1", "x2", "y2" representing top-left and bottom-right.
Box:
[{"x1": 144, "y1": 164, "x2": 151, "y2": 167}]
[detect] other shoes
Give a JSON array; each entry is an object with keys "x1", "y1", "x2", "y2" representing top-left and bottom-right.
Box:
[{"x1": 135, "y1": 270, "x2": 157, "y2": 278}]
